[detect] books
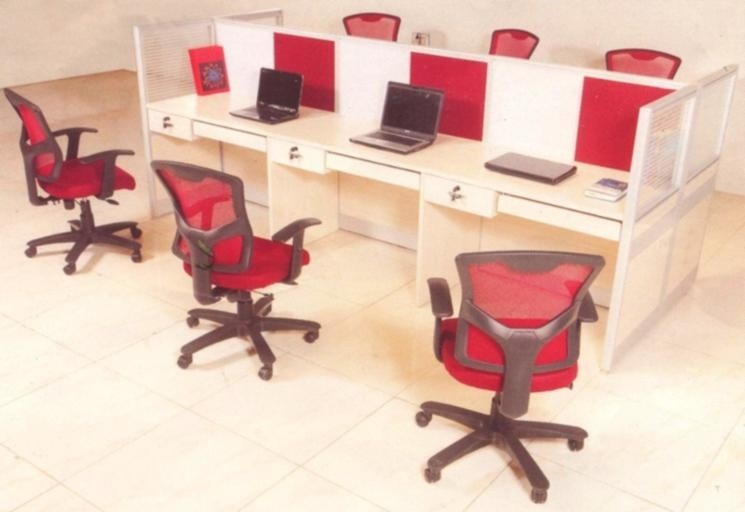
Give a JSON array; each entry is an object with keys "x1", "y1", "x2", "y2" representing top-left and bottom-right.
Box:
[
  {"x1": 585, "y1": 175, "x2": 630, "y2": 203},
  {"x1": 188, "y1": 45, "x2": 231, "y2": 96}
]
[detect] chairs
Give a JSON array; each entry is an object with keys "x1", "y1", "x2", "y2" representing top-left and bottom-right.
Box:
[
  {"x1": 342, "y1": 12, "x2": 404, "y2": 41},
  {"x1": 412, "y1": 248, "x2": 609, "y2": 506},
  {"x1": 482, "y1": 25, "x2": 541, "y2": 61},
  {"x1": 601, "y1": 46, "x2": 684, "y2": 83},
  {"x1": 144, "y1": 158, "x2": 325, "y2": 381},
  {"x1": 4, "y1": 85, "x2": 146, "y2": 277}
]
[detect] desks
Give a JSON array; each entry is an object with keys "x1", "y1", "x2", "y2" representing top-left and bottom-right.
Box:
[{"x1": 124, "y1": 7, "x2": 738, "y2": 382}]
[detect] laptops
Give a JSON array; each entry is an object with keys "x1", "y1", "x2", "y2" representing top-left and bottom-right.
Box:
[
  {"x1": 228, "y1": 66, "x2": 305, "y2": 125},
  {"x1": 349, "y1": 80, "x2": 446, "y2": 155},
  {"x1": 484, "y1": 150, "x2": 578, "y2": 186}
]
